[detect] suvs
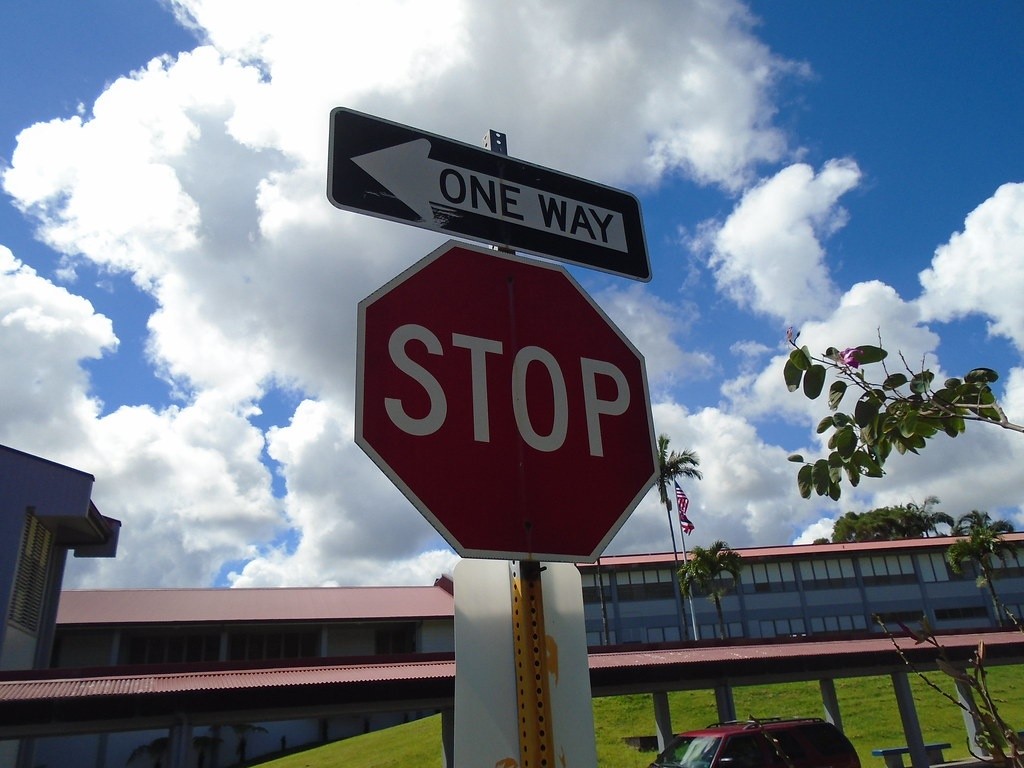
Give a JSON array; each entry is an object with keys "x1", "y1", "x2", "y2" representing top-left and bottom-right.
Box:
[{"x1": 646, "y1": 715, "x2": 862, "y2": 768}]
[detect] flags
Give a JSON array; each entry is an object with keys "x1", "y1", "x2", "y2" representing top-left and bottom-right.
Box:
[{"x1": 675, "y1": 483, "x2": 694, "y2": 536}]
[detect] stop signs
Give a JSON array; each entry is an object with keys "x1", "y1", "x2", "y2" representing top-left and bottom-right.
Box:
[{"x1": 355, "y1": 236, "x2": 662, "y2": 564}]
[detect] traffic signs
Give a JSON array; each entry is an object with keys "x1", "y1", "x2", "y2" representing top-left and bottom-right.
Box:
[{"x1": 327, "y1": 106, "x2": 653, "y2": 283}]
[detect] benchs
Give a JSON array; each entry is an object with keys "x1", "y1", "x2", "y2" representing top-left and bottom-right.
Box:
[{"x1": 872, "y1": 742, "x2": 952, "y2": 768}]
